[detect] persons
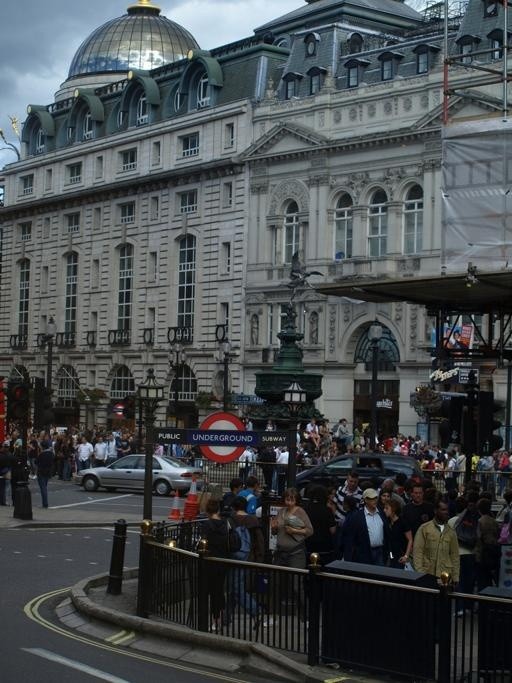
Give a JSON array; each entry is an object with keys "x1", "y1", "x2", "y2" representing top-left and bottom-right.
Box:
[
  {"x1": 443, "y1": 328, "x2": 454, "y2": 349},
  {"x1": 453, "y1": 330, "x2": 464, "y2": 349},
  {"x1": 154, "y1": 419, "x2": 512, "y2": 631},
  {"x1": 1, "y1": 419, "x2": 137, "y2": 508}
]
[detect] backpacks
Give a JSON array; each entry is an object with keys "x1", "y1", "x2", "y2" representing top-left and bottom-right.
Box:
[
  {"x1": 234, "y1": 524, "x2": 252, "y2": 563},
  {"x1": 453, "y1": 460, "x2": 460, "y2": 478}
]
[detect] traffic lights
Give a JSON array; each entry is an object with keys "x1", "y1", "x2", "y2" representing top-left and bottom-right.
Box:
[
  {"x1": 6, "y1": 384, "x2": 27, "y2": 419},
  {"x1": 481, "y1": 393, "x2": 503, "y2": 457}
]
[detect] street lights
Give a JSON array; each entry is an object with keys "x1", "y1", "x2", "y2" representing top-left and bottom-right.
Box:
[
  {"x1": 283, "y1": 380, "x2": 306, "y2": 486},
  {"x1": 167, "y1": 343, "x2": 187, "y2": 429},
  {"x1": 220, "y1": 337, "x2": 230, "y2": 413},
  {"x1": 44, "y1": 316, "x2": 55, "y2": 400},
  {"x1": 138, "y1": 367, "x2": 169, "y2": 519},
  {"x1": 367, "y1": 318, "x2": 383, "y2": 449}
]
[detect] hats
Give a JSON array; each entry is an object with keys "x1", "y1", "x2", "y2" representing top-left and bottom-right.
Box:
[{"x1": 363, "y1": 488, "x2": 378, "y2": 499}]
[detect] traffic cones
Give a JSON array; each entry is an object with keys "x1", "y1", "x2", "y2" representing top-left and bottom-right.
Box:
[
  {"x1": 168, "y1": 490, "x2": 181, "y2": 520},
  {"x1": 183, "y1": 475, "x2": 199, "y2": 520}
]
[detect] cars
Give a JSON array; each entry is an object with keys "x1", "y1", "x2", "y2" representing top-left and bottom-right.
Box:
[
  {"x1": 73, "y1": 453, "x2": 204, "y2": 496},
  {"x1": 297, "y1": 452, "x2": 422, "y2": 490}
]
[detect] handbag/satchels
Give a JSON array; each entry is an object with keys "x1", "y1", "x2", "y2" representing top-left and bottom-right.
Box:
[
  {"x1": 454, "y1": 509, "x2": 482, "y2": 548},
  {"x1": 498, "y1": 524, "x2": 512, "y2": 545},
  {"x1": 229, "y1": 528, "x2": 241, "y2": 552}
]
